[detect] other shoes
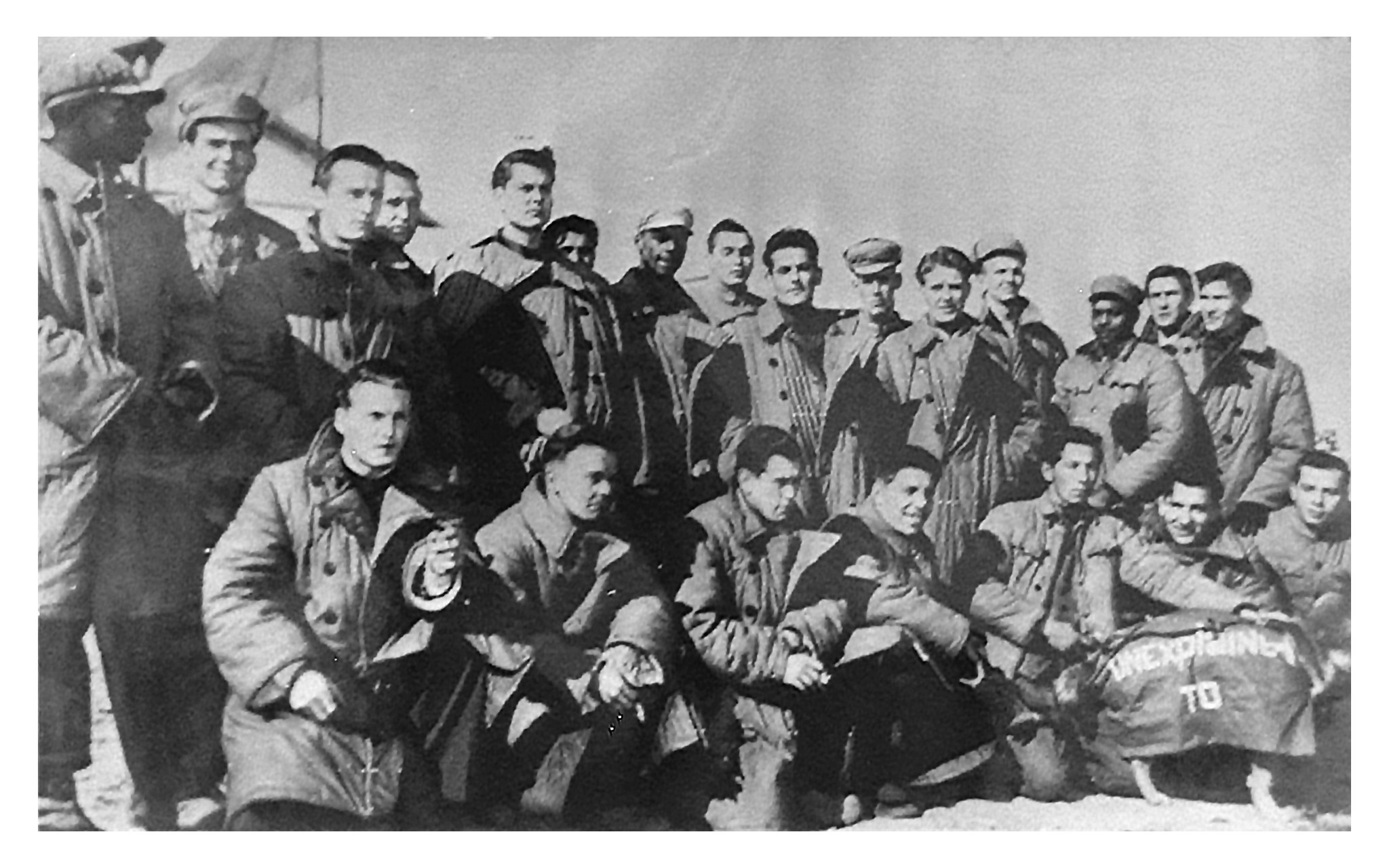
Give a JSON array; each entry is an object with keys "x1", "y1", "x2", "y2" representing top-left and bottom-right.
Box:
[
  {"x1": 176, "y1": 797, "x2": 224, "y2": 829},
  {"x1": 38, "y1": 789, "x2": 102, "y2": 831},
  {"x1": 878, "y1": 802, "x2": 920, "y2": 818},
  {"x1": 801, "y1": 787, "x2": 861, "y2": 828}
]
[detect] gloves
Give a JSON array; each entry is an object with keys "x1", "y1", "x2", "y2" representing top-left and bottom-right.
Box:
[{"x1": 1230, "y1": 499, "x2": 1271, "y2": 539}]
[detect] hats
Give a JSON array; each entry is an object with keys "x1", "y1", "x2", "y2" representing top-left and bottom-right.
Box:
[
  {"x1": 974, "y1": 234, "x2": 1026, "y2": 263},
  {"x1": 635, "y1": 206, "x2": 693, "y2": 236},
  {"x1": 39, "y1": 47, "x2": 167, "y2": 116},
  {"x1": 1088, "y1": 275, "x2": 1144, "y2": 316},
  {"x1": 178, "y1": 89, "x2": 268, "y2": 150},
  {"x1": 844, "y1": 237, "x2": 903, "y2": 275}
]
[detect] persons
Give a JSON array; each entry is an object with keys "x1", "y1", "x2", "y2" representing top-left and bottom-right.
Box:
[{"x1": 39, "y1": 51, "x2": 1351, "y2": 833}]
